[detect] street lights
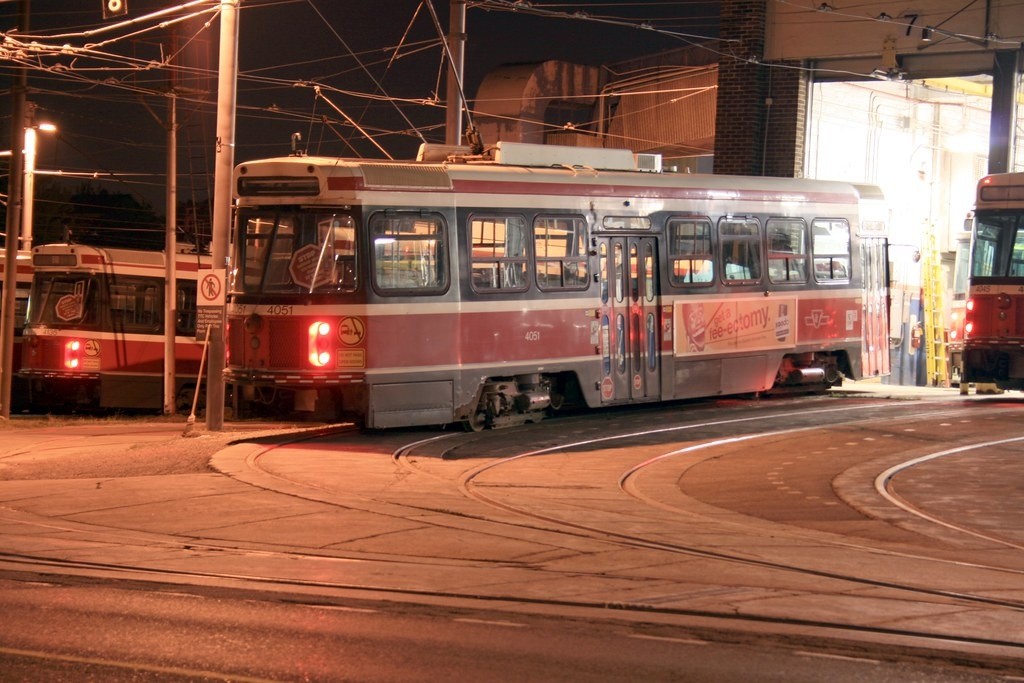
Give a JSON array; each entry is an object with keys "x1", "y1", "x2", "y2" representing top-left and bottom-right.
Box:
[{"x1": 0, "y1": 121, "x2": 60, "y2": 420}]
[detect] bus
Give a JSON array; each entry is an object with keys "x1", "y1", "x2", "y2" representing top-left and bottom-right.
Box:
[
  {"x1": 948, "y1": 170, "x2": 1024, "y2": 392},
  {"x1": 1, "y1": 247, "x2": 38, "y2": 416},
  {"x1": 222, "y1": 2, "x2": 893, "y2": 437},
  {"x1": 18, "y1": 237, "x2": 337, "y2": 422}
]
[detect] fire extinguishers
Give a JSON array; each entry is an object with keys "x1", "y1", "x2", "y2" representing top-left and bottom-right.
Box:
[{"x1": 911, "y1": 321, "x2": 921, "y2": 348}]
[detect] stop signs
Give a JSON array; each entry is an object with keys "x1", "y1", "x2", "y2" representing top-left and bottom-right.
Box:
[
  {"x1": 54, "y1": 294, "x2": 82, "y2": 321},
  {"x1": 288, "y1": 244, "x2": 333, "y2": 290}
]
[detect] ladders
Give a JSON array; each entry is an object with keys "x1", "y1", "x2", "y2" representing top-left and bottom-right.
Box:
[{"x1": 921, "y1": 217, "x2": 948, "y2": 389}]
